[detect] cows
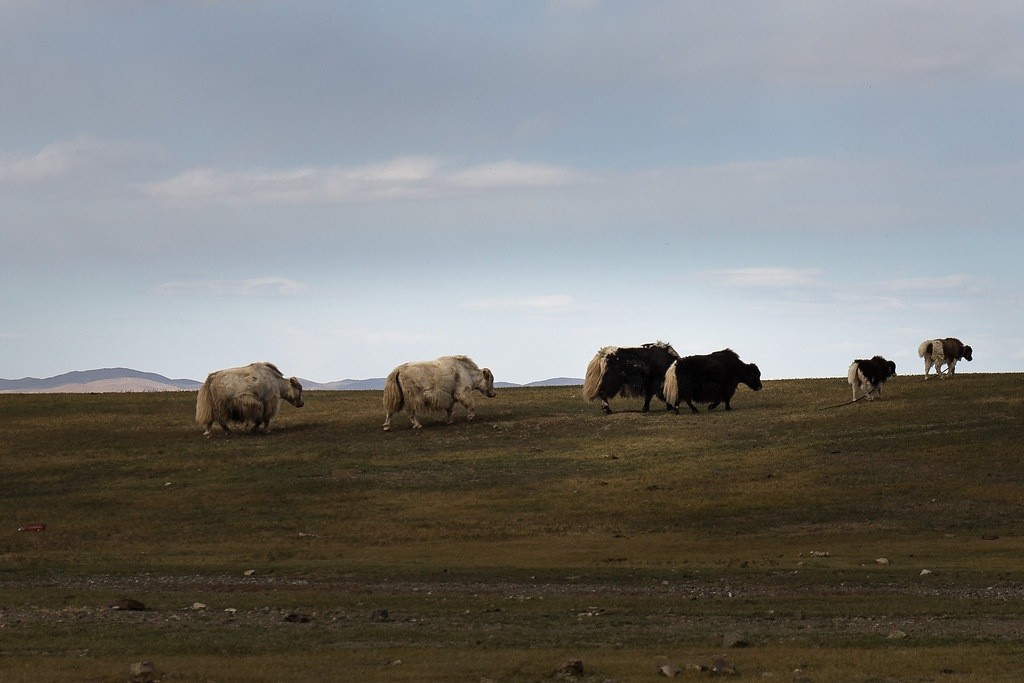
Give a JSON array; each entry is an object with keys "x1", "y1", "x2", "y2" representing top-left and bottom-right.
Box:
[
  {"x1": 583, "y1": 339, "x2": 763, "y2": 415},
  {"x1": 195, "y1": 362, "x2": 304, "y2": 438},
  {"x1": 918, "y1": 337, "x2": 973, "y2": 380},
  {"x1": 847, "y1": 355, "x2": 897, "y2": 402},
  {"x1": 382, "y1": 354, "x2": 498, "y2": 431}
]
